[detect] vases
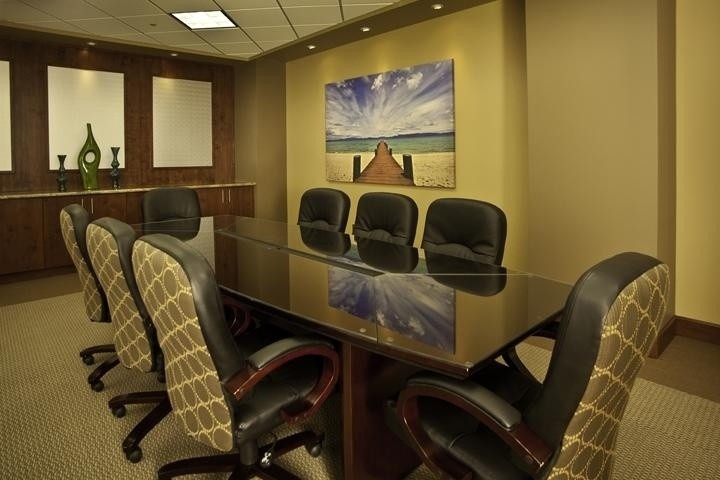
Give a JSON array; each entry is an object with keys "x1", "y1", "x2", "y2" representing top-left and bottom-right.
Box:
[
  {"x1": 57, "y1": 154, "x2": 67, "y2": 192},
  {"x1": 78, "y1": 124, "x2": 101, "y2": 190},
  {"x1": 111, "y1": 146, "x2": 120, "y2": 189}
]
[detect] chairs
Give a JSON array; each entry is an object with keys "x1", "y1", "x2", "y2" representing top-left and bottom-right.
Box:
[
  {"x1": 85, "y1": 217, "x2": 261, "y2": 462},
  {"x1": 132, "y1": 232, "x2": 341, "y2": 480},
  {"x1": 296, "y1": 188, "x2": 351, "y2": 235},
  {"x1": 385, "y1": 252, "x2": 671, "y2": 479},
  {"x1": 59, "y1": 203, "x2": 240, "y2": 392},
  {"x1": 352, "y1": 192, "x2": 419, "y2": 248},
  {"x1": 420, "y1": 198, "x2": 508, "y2": 266}
]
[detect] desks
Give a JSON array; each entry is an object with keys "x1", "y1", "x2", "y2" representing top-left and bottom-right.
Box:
[{"x1": 131, "y1": 214, "x2": 574, "y2": 480}]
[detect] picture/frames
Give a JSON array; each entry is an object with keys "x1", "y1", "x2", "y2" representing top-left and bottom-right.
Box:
[
  {"x1": 1, "y1": 198, "x2": 46, "y2": 277},
  {"x1": 325, "y1": 59, "x2": 456, "y2": 189},
  {"x1": 327, "y1": 232, "x2": 458, "y2": 355}
]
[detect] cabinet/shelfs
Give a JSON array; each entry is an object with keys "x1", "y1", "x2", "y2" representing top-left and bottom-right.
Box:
[
  {"x1": 45, "y1": 194, "x2": 125, "y2": 269},
  {"x1": 192, "y1": 186, "x2": 254, "y2": 231},
  {"x1": 124, "y1": 190, "x2": 143, "y2": 226}
]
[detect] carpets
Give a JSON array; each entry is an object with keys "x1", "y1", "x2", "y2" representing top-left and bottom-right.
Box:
[{"x1": 0, "y1": 286, "x2": 720, "y2": 479}]
